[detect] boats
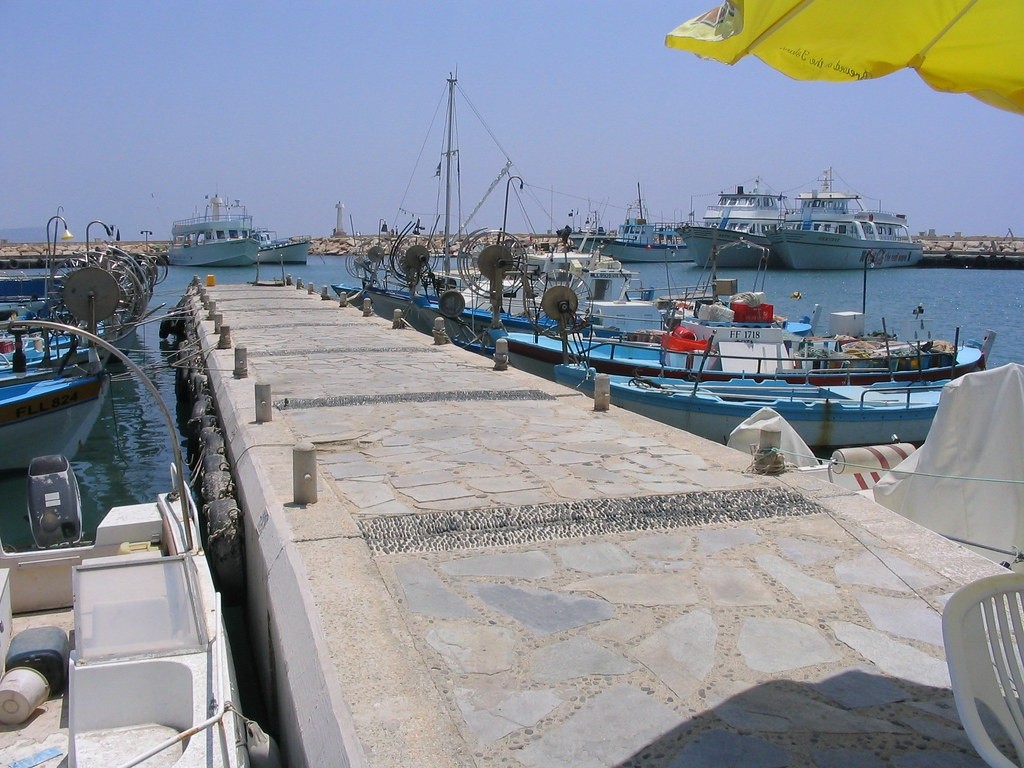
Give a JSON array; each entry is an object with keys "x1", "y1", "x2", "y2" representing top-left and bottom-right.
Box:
[
  {"x1": 165, "y1": 181, "x2": 261, "y2": 267},
  {"x1": 0, "y1": 240, "x2": 157, "y2": 475},
  {"x1": 256, "y1": 225, "x2": 312, "y2": 264},
  {"x1": 0, "y1": 317, "x2": 291, "y2": 768},
  {"x1": 329, "y1": 62, "x2": 997, "y2": 459}
]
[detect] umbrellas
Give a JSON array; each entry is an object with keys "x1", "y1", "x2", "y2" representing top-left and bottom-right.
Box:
[{"x1": 664, "y1": 0, "x2": 1024, "y2": 116}]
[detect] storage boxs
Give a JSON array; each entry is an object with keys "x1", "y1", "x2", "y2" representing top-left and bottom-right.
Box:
[
  {"x1": 694, "y1": 300, "x2": 727, "y2": 318},
  {"x1": 831, "y1": 311, "x2": 864, "y2": 337},
  {"x1": 716, "y1": 279, "x2": 737, "y2": 295},
  {"x1": 731, "y1": 304, "x2": 773, "y2": 322},
  {"x1": 0, "y1": 342, "x2": 14, "y2": 353},
  {"x1": 662, "y1": 334, "x2": 708, "y2": 351}
]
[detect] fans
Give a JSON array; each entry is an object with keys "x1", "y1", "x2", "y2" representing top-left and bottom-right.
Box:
[
  {"x1": 458, "y1": 227, "x2": 528, "y2": 328},
  {"x1": 390, "y1": 235, "x2": 439, "y2": 295},
  {"x1": 360, "y1": 237, "x2": 390, "y2": 282},
  {"x1": 525, "y1": 269, "x2": 593, "y2": 365},
  {"x1": 346, "y1": 247, "x2": 370, "y2": 279},
  {"x1": 47, "y1": 247, "x2": 166, "y2": 376}
]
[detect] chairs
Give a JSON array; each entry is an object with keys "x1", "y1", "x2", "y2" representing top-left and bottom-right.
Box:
[{"x1": 942, "y1": 573, "x2": 1024, "y2": 768}]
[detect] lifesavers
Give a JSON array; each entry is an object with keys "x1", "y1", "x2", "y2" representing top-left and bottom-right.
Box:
[{"x1": 8, "y1": 260, "x2": 19, "y2": 269}]
